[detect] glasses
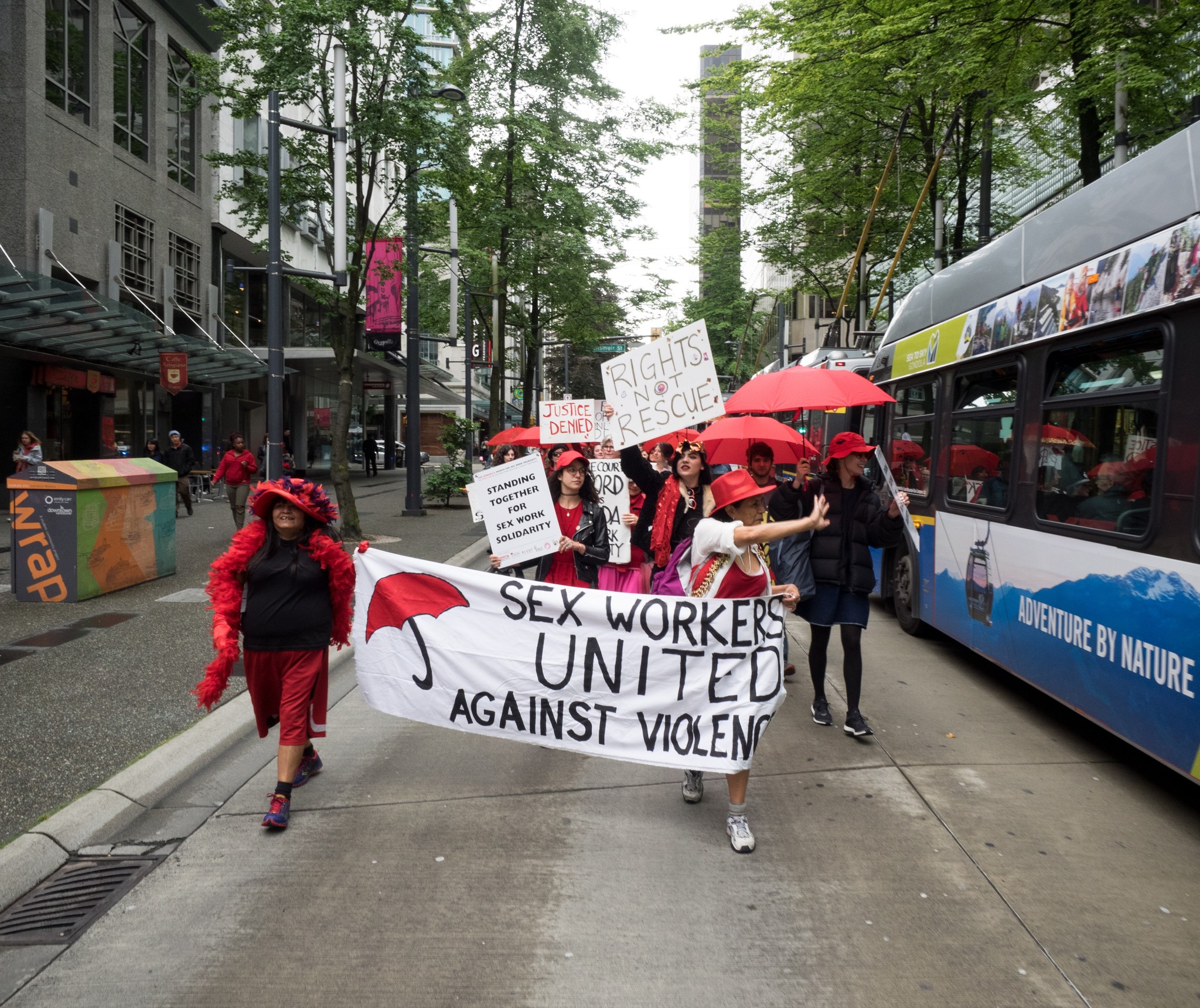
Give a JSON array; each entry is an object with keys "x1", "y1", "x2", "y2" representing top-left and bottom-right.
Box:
[
  {"x1": 1093, "y1": 477, "x2": 1109, "y2": 481},
  {"x1": 551, "y1": 452, "x2": 563, "y2": 457},
  {"x1": 904, "y1": 457, "x2": 914, "y2": 461},
  {"x1": 562, "y1": 467, "x2": 589, "y2": 476},
  {"x1": 603, "y1": 445, "x2": 614, "y2": 451},
  {"x1": 851, "y1": 452, "x2": 870, "y2": 459},
  {"x1": 686, "y1": 488, "x2": 697, "y2": 510}
]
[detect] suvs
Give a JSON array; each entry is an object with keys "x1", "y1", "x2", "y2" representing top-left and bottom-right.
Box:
[{"x1": 375, "y1": 440, "x2": 431, "y2": 466}]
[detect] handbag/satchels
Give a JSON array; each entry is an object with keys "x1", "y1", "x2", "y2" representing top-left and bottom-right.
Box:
[{"x1": 767, "y1": 481, "x2": 825, "y2": 604}]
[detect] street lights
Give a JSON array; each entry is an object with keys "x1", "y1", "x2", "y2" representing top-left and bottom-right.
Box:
[{"x1": 401, "y1": 83, "x2": 466, "y2": 515}]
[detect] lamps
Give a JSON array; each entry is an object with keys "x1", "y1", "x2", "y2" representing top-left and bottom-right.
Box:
[{"x1": 126, "y1": 341, "x2": 142, "y2": 356}]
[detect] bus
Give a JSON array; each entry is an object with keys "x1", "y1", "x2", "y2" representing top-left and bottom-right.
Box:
[
  {"x1": 736, "y1": 105, "x2": 960, "y2": 485},
  {"x1": 859, "y1": 119, "x2": 1200, "y2": 784}
]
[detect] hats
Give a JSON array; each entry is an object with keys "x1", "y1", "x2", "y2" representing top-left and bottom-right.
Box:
[
  {"x1": 821, "y1": 431, "x2": 877, "y2": 465},
  {"x1": 556, "y1": 451, "x2": 590, "y2": 470},
  {"x1": 708, "y1": 468, "x2": 777, "y2": 517},
  {"x1": 903, "y1": 453, "x2": 915, "y2": 459},
  {"x1": 548, "y1": 444, "x2": 568, "y2": 459},
  {"x1": 247, "y1": 476, "x2": 340, "y2": 525}
]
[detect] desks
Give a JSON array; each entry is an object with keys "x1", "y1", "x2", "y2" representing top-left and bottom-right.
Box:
[
  {"x1": 210, "y1": 468, "x2": 231, "y2": 499},
  {"x1": 189, "y1": 470, "x2": 213, "y2": 503}
]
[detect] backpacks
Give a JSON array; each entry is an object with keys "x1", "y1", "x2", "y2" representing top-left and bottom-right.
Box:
[{"x1": 649, "y1": 535, "x2": 720, "y2": 597}]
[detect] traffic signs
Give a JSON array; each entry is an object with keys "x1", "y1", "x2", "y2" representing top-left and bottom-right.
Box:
[{"x1": 586, "y1": 345, "x2": 626, "y2": 352}]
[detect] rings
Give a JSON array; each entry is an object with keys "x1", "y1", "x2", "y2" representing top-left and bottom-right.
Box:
[
  {"x1": 792, "y1": 602, "x2": 796, "y2": 607},
  {"x1": 792, "y1": 596, "x2": 796, "y2": 601}
]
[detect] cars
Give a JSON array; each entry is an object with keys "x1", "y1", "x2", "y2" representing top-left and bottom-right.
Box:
[{"x1": 370, "y1": 443, "x2": 396, "y2": 464}]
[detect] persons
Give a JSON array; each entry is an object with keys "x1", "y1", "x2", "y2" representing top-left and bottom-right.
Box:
[
  {"x1": 889, "y1": 416, "x2": 1199, "y2": 564},
  {"x1": 770, "y1": 431, "x2": 911, "y2": 737},
  {"x1": 191, "y1": 455, "x2": 213, "y2": 495},
  {"x1": 474, "y1": 361, "x2": 897, "y2": 596},
  {"x1": 679, "y1": 469, "x2": 831, "y2": 854},
  {"x1": 220, "y1": 478, "x2": 347, "y2": 828},
  {"x1": 209, "y1": 432, "x2": 258, "y2": 531},
  {"x1": 5, "y1": 430, "x2": 43, "y2": 521},
  {"x1": 963, "y1": 225, "x2": 1200, "y2": 356},
  {"x1": 162, "y1": 430, "x2": 195, "y2": 517},
  {"x1": 143, "y1": 436, "x2": 166, "y2": 465},
  {"x1": 256, "y1": 416, "x2": 379, "y2": 481}
]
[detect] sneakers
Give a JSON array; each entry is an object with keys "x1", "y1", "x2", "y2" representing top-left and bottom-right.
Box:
[
  {"x1": 681, "y1": 769, "x2": 703, "y2": 803},
  {"x1": 810, "y1": 697, "x2": 833, "y2": 726},
  {"x1": 261, "y1": 793, "x2": 291, "y2": 827},
  {"x1": 844, "y1": 707, "x2": 874, "y2": 736},
  {"x1": 292, "y1": 750, "x2": 323, "y2": 788},
  {"x1": 725, "y1": 813, "x2": 756, "y2": 853}
]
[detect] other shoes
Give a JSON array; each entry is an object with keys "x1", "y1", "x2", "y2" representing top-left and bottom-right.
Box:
[{"x1": 187, "y1": 506, "x2": 194, "y2": 515}]
